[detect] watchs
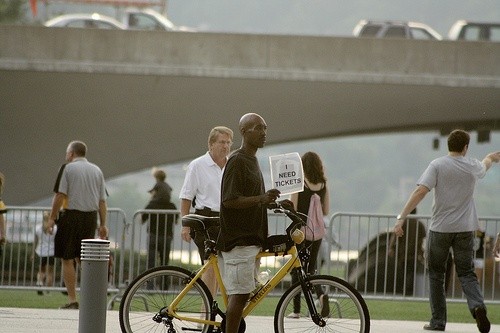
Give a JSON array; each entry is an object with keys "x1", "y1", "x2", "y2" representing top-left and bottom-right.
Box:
[{"x1": 396, "y1": 214, "x2": 404, "y2": 220}]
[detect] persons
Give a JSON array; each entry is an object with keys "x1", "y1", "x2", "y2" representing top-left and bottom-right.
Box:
[
  {"x1": 0, "y1": 163, "x2": 500, "y2": 295},
  {"x1": 45, "y1": 140, "x2": 109, "y2": 310},
  {"x1": 287, "y1": 152, "x2": 331, "y2": 318},
  {"x1": 177, "y1": 126, "x2": 234, "y2": 328},
  {"x1": 393, "y1": 129, "x2": 500, "y2": 333},
  {"x1": 218, "y1": 113, "x2": 295, "y2": 333}
]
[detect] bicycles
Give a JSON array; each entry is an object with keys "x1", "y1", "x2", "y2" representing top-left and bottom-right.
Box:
[{"x1": 119, "y1": 200, "x2": 371, "y2": 333}]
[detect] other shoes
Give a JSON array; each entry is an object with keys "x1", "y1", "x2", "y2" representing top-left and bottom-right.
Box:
[
  {"x1": 320, "y1": 294, "x2": 330, "y2": 318},
  {"x1": 36, "y1": 281, "x2": 44, "y2": 295},
  {"x1": 473, "y1": 306, "x2": 491, "y2": 333},
  {"x1": 423, "y1": 323, "x2": 445, "y2": 331},
  {"x1": 60, "y1": 301, "x2": 78, "y2": 309},
  {"x1": 286, "y1": 312, "x2": 300, "y2": 318}
]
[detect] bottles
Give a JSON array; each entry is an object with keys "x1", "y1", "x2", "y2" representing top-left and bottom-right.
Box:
[{"x1": 248, "y1": 269, "x2": 271, "y2": 302}]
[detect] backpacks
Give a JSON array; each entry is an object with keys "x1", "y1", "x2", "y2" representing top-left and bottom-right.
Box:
[{"x1": 301, "y1": 179, "x2": 324, "y2": 241}]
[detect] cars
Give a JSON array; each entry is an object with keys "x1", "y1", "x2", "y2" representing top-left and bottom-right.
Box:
[
  {"x1": 44, "y1": 8, "x2": 198, "y2": 32},
  {"x1": 352, "y1": 18, "x2": 444, "y2": 41},
  {"x1": 447, "y1": 19, "x2": 500, "y2": 44}
]
